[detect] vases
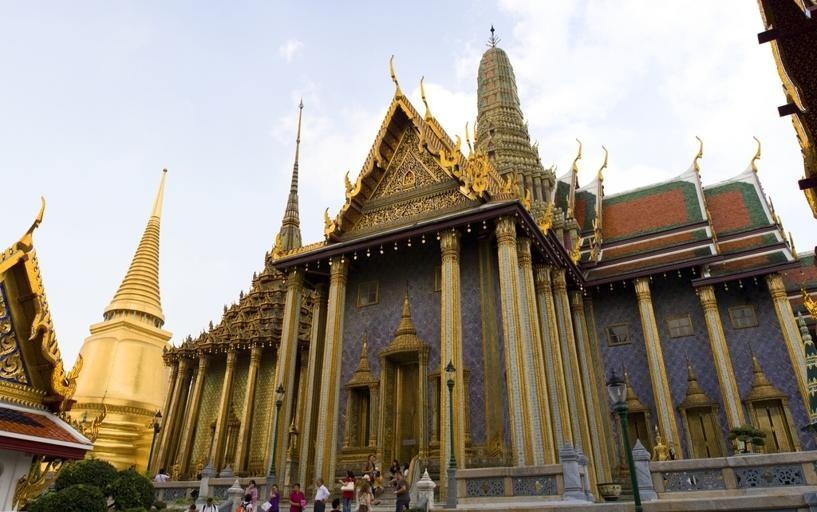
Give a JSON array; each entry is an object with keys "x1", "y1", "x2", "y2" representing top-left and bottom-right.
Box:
[{"x1": 594, "y1": 480, "x2": 624, "y2": 502}]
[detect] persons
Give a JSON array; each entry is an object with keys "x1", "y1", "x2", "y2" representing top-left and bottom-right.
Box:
[
  {"x1": 153, "y1": 454, "x2": 410, "y2": 511},
  {"x1": 668, "y1": 447, "x2": 692, "y2": 490}
]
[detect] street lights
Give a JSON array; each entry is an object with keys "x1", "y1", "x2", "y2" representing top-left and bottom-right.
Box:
[
  {"x1": 265, "y1": 382, "x2": 285, "y2": 502},
  {"x1": 604, "y1": 367, "x2": 643, "y2": 511},
  {"x1": 144, "y1": 410, "x2": 162, "y2": 474},
  {"x1": 441, "y1": 360, "x2": 460, "y2": 509}
]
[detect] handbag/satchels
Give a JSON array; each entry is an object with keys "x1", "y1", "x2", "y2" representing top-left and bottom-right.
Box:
[
  {"x1": 341, "y1": 482, "x2": 354, "y2": 491},
  {"x1": 261, "y1": 501, "x2": 272, "y2": 512},
  {"x1": 236, "y1": 502, "x2": 248, "y2": 512}
]
